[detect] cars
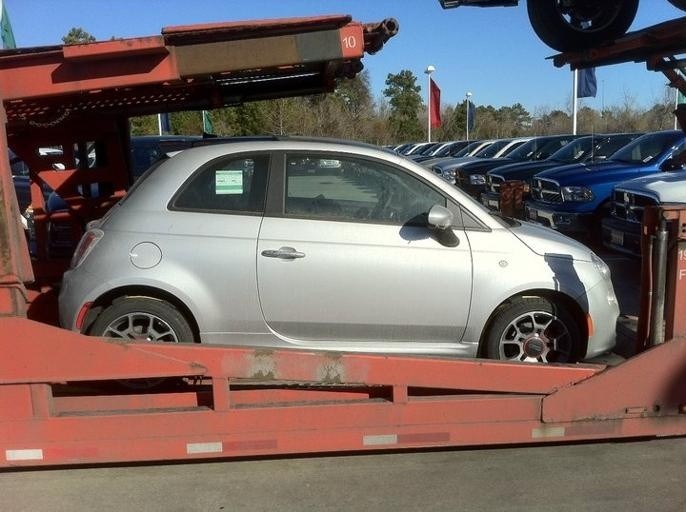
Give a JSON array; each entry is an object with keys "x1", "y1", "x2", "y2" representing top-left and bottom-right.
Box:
[
  {"x1": 439, "y1": 1, "x2": 685, "y2": 53},
  {"x1": 286, "y1": 156, "x2": 342, "y2": 175},
  {"x1": 131, "y1": 135, "x2": 201, "y2": 181},
  {"x1": 54, "y1": 135, "x2": 623, "y2": 390},
  {"x1": 38, "y1": 147, "x2": 97, "y2": 171}
]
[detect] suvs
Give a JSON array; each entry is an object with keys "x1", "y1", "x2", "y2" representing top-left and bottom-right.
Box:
[
  {"x1": 480, "y1": 130, "x2": 645, "y2": 220},
  {"x1": 523, "y1": 130, "x2": 686, "y2": 241},
  {"x1": 600, "y1": 171, "x2": 686, "y2": 256},
  {"x1": 346, "y1": 129, "x2": 584, "y2": 199}
]
